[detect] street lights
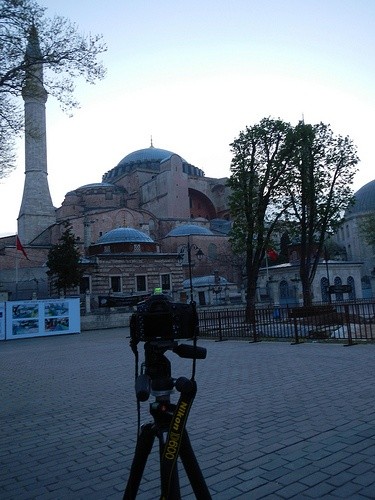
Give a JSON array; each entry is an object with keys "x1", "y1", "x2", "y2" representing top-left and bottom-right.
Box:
[
  {"x1": 325, "y1": 248, "x2": 340, "y2": 302},
  {"x1": 175, "y1": 243, "x2": 204, "y2": 306}
]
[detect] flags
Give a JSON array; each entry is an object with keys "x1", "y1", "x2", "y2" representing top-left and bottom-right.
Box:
[
  {"x1": 17, "y1": 235, "x2": 31, "y2": 262},
  {"x1": 266, "y1": 244, "x2": 280, "y2": 262}
]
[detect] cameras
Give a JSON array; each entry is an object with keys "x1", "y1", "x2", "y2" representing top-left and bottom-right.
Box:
[{"x1": 130, "y1": 293, "x2": 199, "y2": 342}]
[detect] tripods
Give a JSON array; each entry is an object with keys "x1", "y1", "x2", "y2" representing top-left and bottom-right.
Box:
[{"x1": 122, "y1": 343, "x2": 213, "y2": 500}]
